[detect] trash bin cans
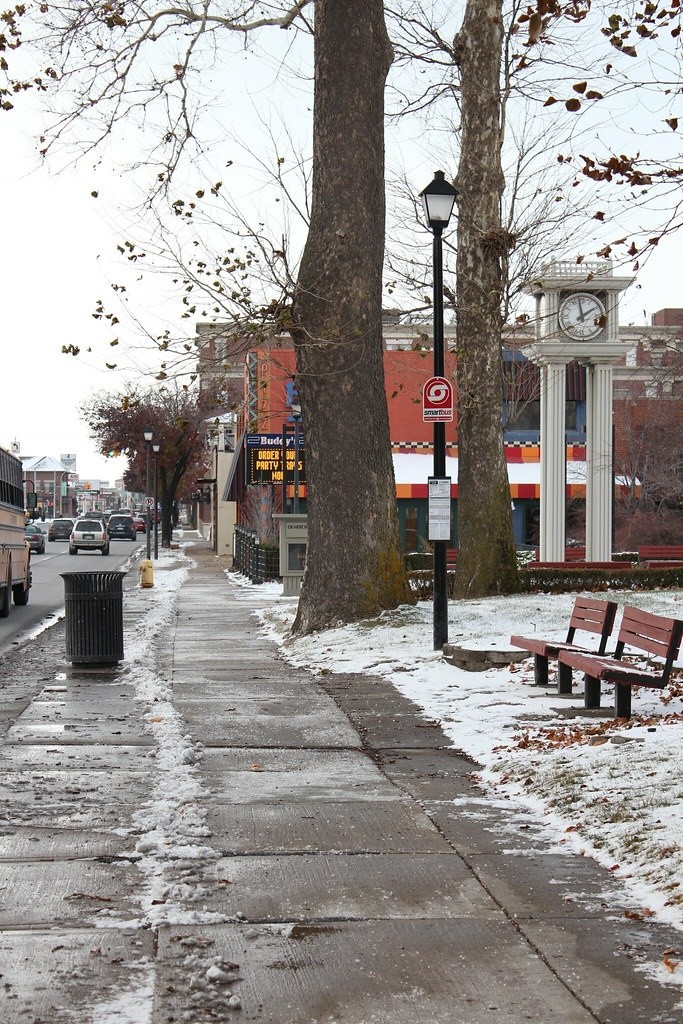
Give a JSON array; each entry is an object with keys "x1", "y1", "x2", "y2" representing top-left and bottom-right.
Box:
[{"x1": 59, "y1": 569, "x2": 129, "y2": 667}]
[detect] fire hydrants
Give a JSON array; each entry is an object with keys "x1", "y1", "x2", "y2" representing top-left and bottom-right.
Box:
[{"x1": 139, "y1": 558, "x2": 154, "y2": 588}]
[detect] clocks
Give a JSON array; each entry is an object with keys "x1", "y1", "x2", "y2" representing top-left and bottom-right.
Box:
[{"x1": 559, "y1": 292, "x2": 605, "y2": 341}]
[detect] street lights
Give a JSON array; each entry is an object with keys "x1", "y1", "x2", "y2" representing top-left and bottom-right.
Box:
[
  {"x1": 142, "y1": 424, "x2": 155, "y2": 561},
  {"x1": 289, "y1": 397, "x2": 301, "y2": 513},
  {"x1": 150, "y1": 438, "x2": 162, "y2": 560},
  {"x1": 418, "y1": 169, "x2": 460, "y2": 653}
]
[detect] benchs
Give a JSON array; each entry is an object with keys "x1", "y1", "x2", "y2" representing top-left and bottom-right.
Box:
[
  {"x1": 446, "y1": 545, "x2": 682, "y2": 571},
  {"x1": 509, "y1": 595, "x2": 618, "y2": 696},
  {"x1": 558, "y1": 606, "x2": 682, "y2": 718}
]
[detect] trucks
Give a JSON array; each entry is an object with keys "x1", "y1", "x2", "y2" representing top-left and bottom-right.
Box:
[{"x1": 0, "y1": 446, "x2": 39, "y2": 619}]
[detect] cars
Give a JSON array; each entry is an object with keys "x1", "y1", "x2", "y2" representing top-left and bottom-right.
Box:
[
  {"x1": 105, "y1": 513, "x2": 137, "y2": 542},
  {"x1": 69, "y1": 518, "x2": 111, "y2": 555},
  {"x1": 79, "y1": 506, "x2": 160, "y2": 531},
  {"x1": 47, "y1": 519, "x2": 75, "y2": 542},
  {"x1": 25, "y1": 523, "x2": 48, "y2": 554}
]
